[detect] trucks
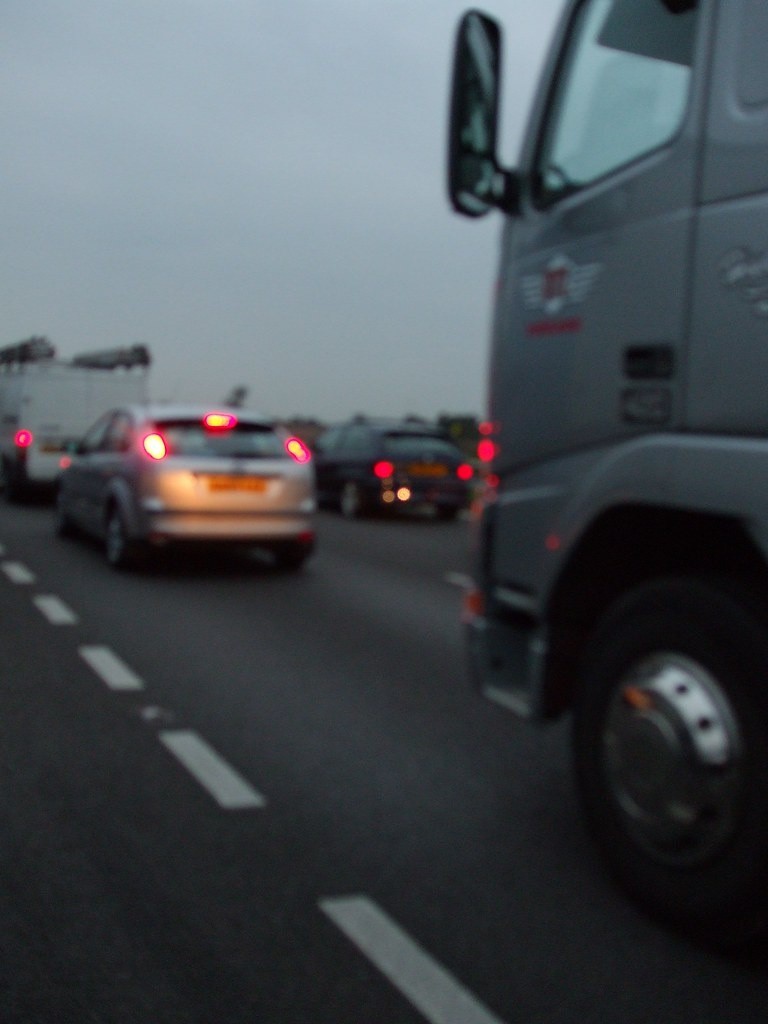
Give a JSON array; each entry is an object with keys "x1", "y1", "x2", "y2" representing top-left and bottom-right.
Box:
[
  {"x1": 0, "y1": 335, "x2": 149, "y2": 497},
  {"x1": 446, "y1": 0, "x2": 768, "y2": 937}
]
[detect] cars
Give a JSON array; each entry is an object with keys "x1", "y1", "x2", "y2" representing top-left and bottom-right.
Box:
[
  {"x1": 310, "y1": 420, "x2": 470, "y2": 521},
  {"x1": 54, "y1": 404, "x2": 317, "y2": 568}
]
